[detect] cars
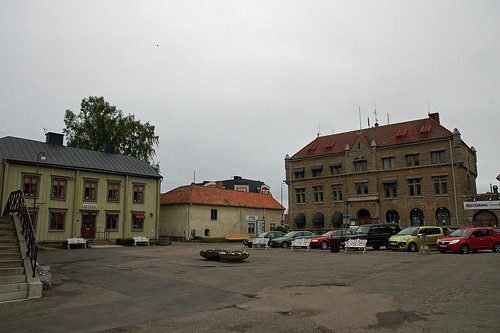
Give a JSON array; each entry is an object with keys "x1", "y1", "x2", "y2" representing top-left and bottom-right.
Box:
[
  {"x1": 442, "y1": 225, "x2": 460, "y2": 236},
  {"x1": 268, "y1": 230, "x2": 318, "y2": 249},
  {"x1": 436, "y1": 227, "x2": 500, "y2": 255},
  {"x1": 309, "y1": 230, "x2": 350, "y2": 250},
  {"x1": 243, "y1": 231, "x2": 286, "y2": 248},
  {"x1": 387, "y1": 225, "x2": 444, "y2": 252}
]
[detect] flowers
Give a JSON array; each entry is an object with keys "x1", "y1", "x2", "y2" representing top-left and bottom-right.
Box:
[{"x1": 200, "y1": 249, "x2": 250, "y2": 256}]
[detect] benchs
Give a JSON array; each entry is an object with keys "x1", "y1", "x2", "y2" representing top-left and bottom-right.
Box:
[
  {"x1": 252, "y1": 238, "x2": 269, "y2": 250},
  {"x1": 345, "y1": 238, "x2": 367, "y2": 254},
  {"x1": 133, "y1": 237, "x2": 150, "y2": 247},
  {"x1": 66, "y1": 237, "x2": 86, "y2": 250},
  {"x1": 291, "y1": 239, "x2": 311, "y2": 252}
]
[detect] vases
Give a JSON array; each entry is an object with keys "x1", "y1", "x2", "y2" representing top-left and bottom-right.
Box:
[{"x1": 202, "y1": 255, "x2": 249, "y2": 261}]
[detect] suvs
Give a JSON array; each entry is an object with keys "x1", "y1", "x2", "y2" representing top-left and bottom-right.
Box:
[{"x1": 340, "y1": 223, "x2": 401, "y2": 251}]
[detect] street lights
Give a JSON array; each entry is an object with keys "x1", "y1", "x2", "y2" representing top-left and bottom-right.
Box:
[{"x1": 32, "y1": 151, "x2": 47, "y2": 229}]
